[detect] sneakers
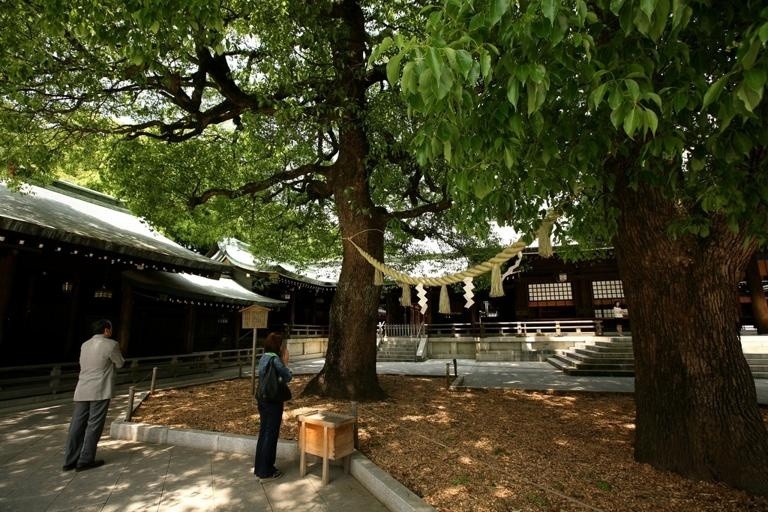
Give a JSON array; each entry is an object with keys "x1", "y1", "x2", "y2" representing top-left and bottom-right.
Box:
[
  {"x1": 254, "y1": 473, "x2": 282, "y2": 483},
  {"x1": 62, "y1": 459, "x2": 105, "y2": 472}
]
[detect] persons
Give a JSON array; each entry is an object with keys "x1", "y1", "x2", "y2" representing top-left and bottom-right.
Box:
[
  {"x1": 62, "y1": 319, "x2": 125, "y2": 472},
  {"x1": 254, "y1": 334, "x2": 293, "y2": 483}
]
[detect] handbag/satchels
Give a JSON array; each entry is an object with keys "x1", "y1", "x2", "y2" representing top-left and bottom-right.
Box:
[{"x1": 255, "y1": 365, "x2": 292, "y2": 402}]
